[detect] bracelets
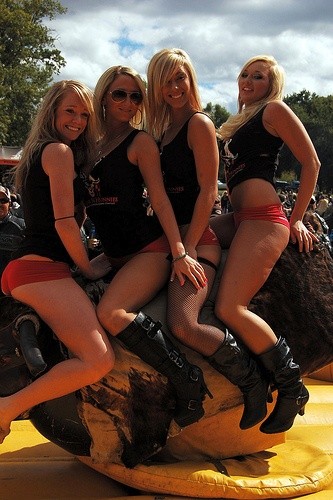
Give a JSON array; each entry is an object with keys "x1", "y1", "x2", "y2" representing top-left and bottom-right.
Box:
[{"x1": 169, "y1": 252, "x2": 189, "y2": 263}]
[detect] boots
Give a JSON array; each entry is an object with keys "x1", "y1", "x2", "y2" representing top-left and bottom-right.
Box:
[
  {"x1": 258, "y1": 337, "x2": 308, "y2": 434},
  {"x1": 114, "y1": 312, "x2": 218, "y2": 428},
  {"x1": 204, "y1": 328, "x2": 275, "y2": 430}
]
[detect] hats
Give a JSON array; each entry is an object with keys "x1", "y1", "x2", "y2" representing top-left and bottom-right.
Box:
[{"x1": 0, "y1": 184, "x2": 12, "y2": 204}]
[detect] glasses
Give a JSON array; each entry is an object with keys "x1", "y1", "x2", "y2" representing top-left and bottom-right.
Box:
[
  {"x1": 105, "y1": 87, "x2": 144, "y2": 106},
  {"x1": 0, "y1": 196, "x2": 9, "y2": 203}
]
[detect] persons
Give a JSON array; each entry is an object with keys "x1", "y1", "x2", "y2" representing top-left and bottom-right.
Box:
[
  {"x1": 0, "y1": 80, "x2": 116, "y2": 445},
  {"x1": 0, "y1": 178, "x2": 333, "y2": 263},
  {"x1": 213, "y1": 52, "x2": 322, "y2": 434},
  {"x1": 77, "y1": 64, "x2": 215, "y2": 428},
  {"x1": 146, "y1": 48, "x2": 280, "y2": 431}
]
